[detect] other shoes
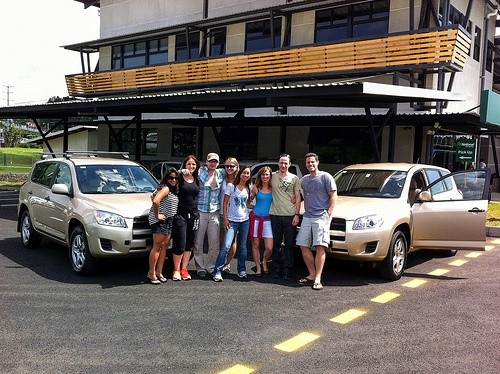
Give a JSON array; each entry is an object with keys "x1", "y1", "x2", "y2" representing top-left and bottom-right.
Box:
[
  {"x1": 256, "y1": 272, "x2": 261, "y2": 277},
  {"x1": 196, "y1": 268, "x2": 206, "y2": 277},
  {"x1": 263, "y1": 268, "x2": 269, "y2": 275},
  {"x1": 181, "y1": 269, "x2": 191, "y2": 280},
  {"x1": 273, "y1": 275, "x2": 281, "y2": 278},
  {"x1": 173, "y1": 270, "x2": 181, "y2": 281},
  {"x1": 282, "y1": 275, "x2": 287, "y2": 280},
  {"x1": 212, "y1": 271, "x2": 223, "y2": 282},
  {"x1": 224, "y1": 264, "x2": 231, "y2": 273},
  {"x1": 238, "y1": 271, "x2": 248, "y2": 279},
  {"x1": 207, "y1": 268, "x2": 214, "y2": 273}
]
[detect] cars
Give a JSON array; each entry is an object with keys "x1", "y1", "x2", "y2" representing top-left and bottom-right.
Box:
[
  {"x1": 148, "y1": 157, "x2": 491, "y2": 282},
  {"x1": 13, "y1": 138, "x2": 35, "y2": 147}
]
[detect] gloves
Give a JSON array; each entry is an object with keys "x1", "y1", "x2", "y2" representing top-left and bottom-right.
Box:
[{"x1": 181, "y1": 169, "x2": 190, "y2": 175}]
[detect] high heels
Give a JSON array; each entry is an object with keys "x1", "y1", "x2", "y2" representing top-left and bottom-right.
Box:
[
  {"x1": 156, "y1": 273, "x2": 168, "y2": 283},
  {"x1": 146, "y1": 273, "x2": 161, "y2": 285}
]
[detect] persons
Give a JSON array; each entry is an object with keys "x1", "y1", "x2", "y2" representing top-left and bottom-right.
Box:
[
  {"x1": 146, "y1": 153, "x2": 337, "y2": 290},
  {"x1": 479, "y1": 159, "x2": 486, "y2": 169}
]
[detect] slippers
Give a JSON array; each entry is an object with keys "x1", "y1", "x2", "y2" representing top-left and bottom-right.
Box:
[
  {"x1": 298, "y1": 277, "x2": 314, "y2": 283},
  {"x1": 313, "y1": 282, "x2": 323, "y2": 290}
]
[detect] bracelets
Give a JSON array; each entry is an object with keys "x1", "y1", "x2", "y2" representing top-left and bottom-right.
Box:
[{"x1": 295, "y1": 214, "x2": 299, "y2": 216}]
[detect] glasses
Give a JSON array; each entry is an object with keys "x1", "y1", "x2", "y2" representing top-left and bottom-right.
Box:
[
  {"x1": 208, "y1": 160, "x2": 218, "y2": 164},
  {"x1": 168, "y1": 176, "x2": 179, "y2": 180},
  {"x1": 225, "y1": 164, "x2": 235, "y2": 169}
]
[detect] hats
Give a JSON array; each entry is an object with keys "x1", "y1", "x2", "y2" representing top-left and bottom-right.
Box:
[{"x1": 206, "y1": 152, "x2": 219, "y2": 162}]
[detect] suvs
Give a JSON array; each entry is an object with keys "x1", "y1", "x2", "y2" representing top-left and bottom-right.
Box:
[{"x1": 17, "y1": 150, "x2": 199, "y2": 275}]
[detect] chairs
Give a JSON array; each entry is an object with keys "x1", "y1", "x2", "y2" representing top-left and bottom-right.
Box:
[{"x1": 409, "y1": 177, "x2": 417, "y2": 204}]
